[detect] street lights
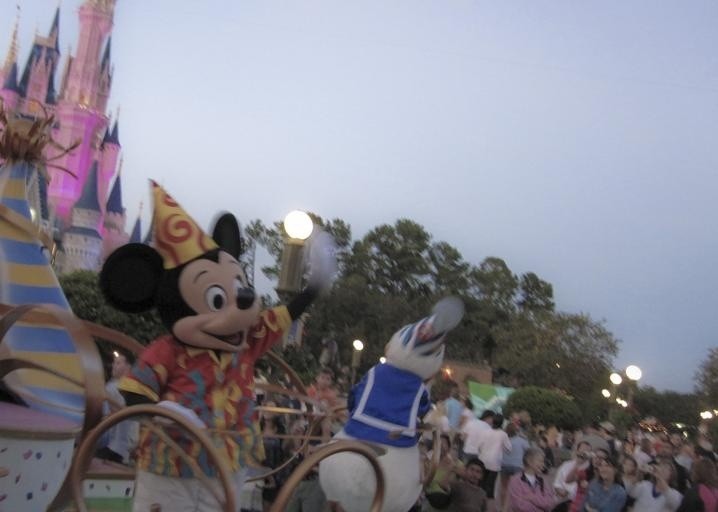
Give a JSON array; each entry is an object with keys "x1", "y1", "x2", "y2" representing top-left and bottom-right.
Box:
[
  {"x1": 344, "y1": 333, "x2": 367, "y2": 400},
  {"x1": 597, "y1": 361, "x2": 646, "y2": 432},
  {"x1": 265, "y1": 209, "x2": 320, "y2": 399}
]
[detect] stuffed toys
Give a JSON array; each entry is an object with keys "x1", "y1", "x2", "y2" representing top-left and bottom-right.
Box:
[
  {"x1": 317, "y1": 293, "x2": 465, "y2": 511},
  {"x1": 98, "y1": 177, "x2": 341, "y2": 512}
]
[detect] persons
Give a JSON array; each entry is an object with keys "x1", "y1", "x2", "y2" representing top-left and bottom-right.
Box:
[
  {"x1": 94, "y1": 352, "x2": 142, "y2": 463},
  {"x1": 414, "y1": 380, "x2": 716, "y2": 512},
  {"x1": 250, "y1": 363, "x2": 350, "y2": 487}
]
